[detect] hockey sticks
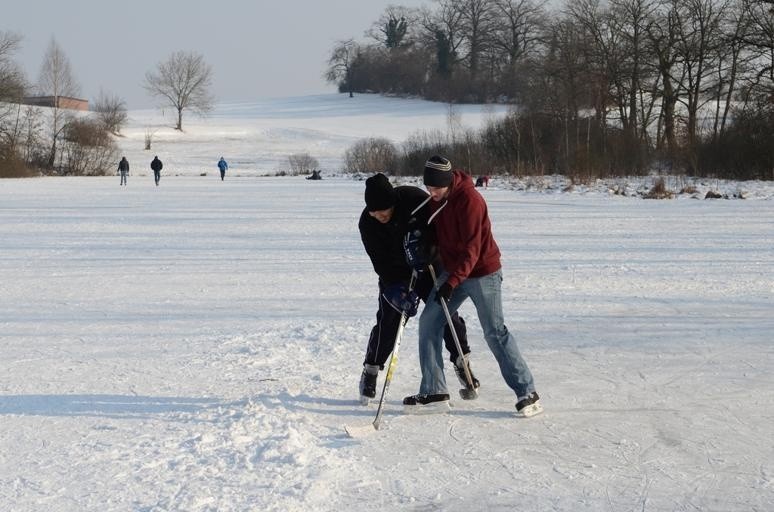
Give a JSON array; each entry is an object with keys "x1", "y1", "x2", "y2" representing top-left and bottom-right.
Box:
[
  {"x1": 428, "y1": 263, "x2": 478, "y2": 399},
  {"x1": 345, "y1": 269, "x2": 418, "y2": 438}
]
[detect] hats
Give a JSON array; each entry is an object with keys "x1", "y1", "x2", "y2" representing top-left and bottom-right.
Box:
[
  {"x1": 423, "y1": 153, "x2": 452, "y2": 187},
  {"x1": 365, "y1": 173, "x2": 395, "y2": 211}
]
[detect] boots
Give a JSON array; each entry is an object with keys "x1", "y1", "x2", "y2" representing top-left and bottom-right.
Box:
[
  {"x1": 359, "y1": 371, "x2": 378, "y2": 398},
  {"x1": 453, "y1": 364, "x2": 480, "y2": 389},
  {"x1": 403, "y1": 392, "x2": 450, "y2": 405},
  {"x1": 515, "y1": 392, "x2": 540, "y2": 411}
]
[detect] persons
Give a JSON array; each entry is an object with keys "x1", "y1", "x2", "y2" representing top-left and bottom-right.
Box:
[
  {"x1": 474, "y1": 176, "x2": 483, "y2": 187},
  {"x1": 304, "y1": 170, "x2": 321, "y2": 181},
  {"x1": 216, "y1": 157, "x2": 227, "y2": 181},
  {"x1": 480, "y1": 176, "x2": 489, "y2": 188},
  {"x1": 117, "y1": 156, "x2": 128, "y2": 186},
  {"x1": 150, "y1": 155, "x2": 162, "y2": 185},
  {"x1": 401, "y1": 153, "x2": 543, "y2": 412},
  {"x1": 353, "y1": 172, "x2": 481, "y2": 398}
]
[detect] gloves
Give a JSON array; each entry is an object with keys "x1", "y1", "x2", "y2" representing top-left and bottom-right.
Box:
[
  {"x1": 385, "y1": 286, "x2": 419, "y2": 317},
  {"x1": 421, "y1": 266, "x2": 440, "y2": 281},
  {"x1": 433, "y1": 282, "x2": 453, "y2": 306}
]
